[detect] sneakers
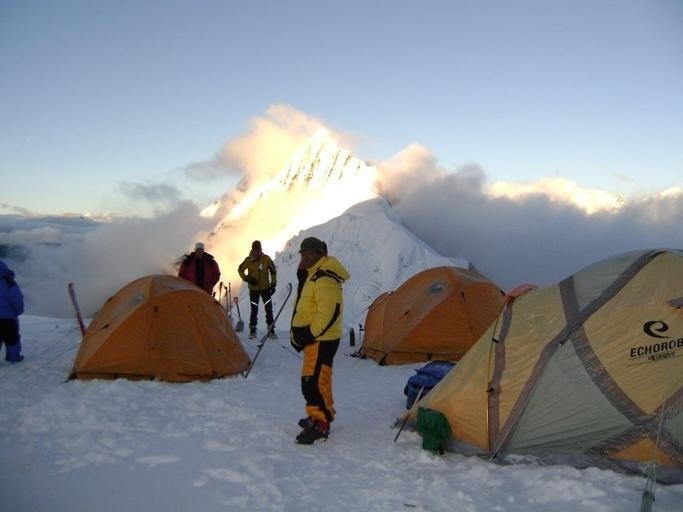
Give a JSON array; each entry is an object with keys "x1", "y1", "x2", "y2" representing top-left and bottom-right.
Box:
[
  {"x1": 296, "y1": 424, "x2": 330, "y2": 445},
  {"x1": 5, "y1": 354, "x2": 25, "y2": 362},
  {"x1": 298, "y1": 416, "x2": 311, "y2": 428}
]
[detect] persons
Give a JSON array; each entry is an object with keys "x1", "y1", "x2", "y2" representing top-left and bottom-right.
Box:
[
  {"x1": 0, "y1": 260, "x2": 24, "y2": 362},
  {"x1": 288, "y1": 237, "x2": 351, "y2": 444},
  {"x1": 177, "y1": 243, "x2": 219, "y2": 294},
  {"x1": 237, "y1": 240, "x2": 279, "y2": 339}
]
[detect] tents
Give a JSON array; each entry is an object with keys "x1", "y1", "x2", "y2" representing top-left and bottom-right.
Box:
[
  {"x1": 393, "y1": 247, "x2": 681, "y2": 485},
  {"x1": 360, "y1": 265, "x2": 502, "y2": 366},
  {"x1": 70, "y1": 273, "x2": 252, "y2": 383}
]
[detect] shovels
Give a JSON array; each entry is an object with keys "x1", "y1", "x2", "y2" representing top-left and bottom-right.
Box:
[{"x1": 234, "y1": 297, "x2": 243, "y2": 331}]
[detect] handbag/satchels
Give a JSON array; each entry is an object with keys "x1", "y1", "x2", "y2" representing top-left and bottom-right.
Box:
[{"x1": 403, "y1": 360, "x2": 457, "y2": 410}]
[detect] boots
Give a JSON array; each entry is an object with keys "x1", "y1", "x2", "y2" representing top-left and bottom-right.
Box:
[
  {"x1": 267, "y1": 324, "x2": 279, "y2": 340},
  {"x1": 248, "y1": 325, "x2": 258, "y2": 339}
]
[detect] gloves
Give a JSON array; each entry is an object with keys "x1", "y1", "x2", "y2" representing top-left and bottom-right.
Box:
[
  {"x1": 244, "y1": 275, "x2": 259, "y2": 286},
  {"x1": 265, "y1": 286, "x2": 275, "y2": 296}
]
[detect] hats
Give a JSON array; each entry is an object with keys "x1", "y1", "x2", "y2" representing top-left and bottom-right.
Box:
[
  {"x1": 298, "y1": 237, "x2": 325, "y2": 254},
  {"x1": 195, "y1": 242, "x2": 205, "y2": 250}
]
